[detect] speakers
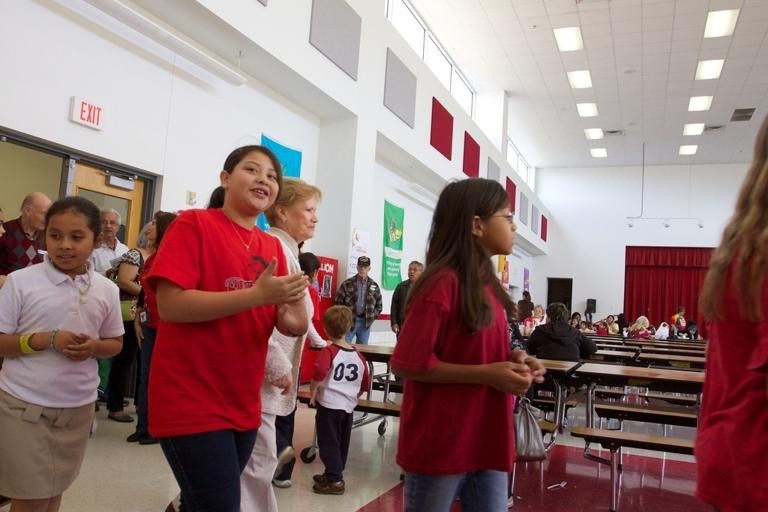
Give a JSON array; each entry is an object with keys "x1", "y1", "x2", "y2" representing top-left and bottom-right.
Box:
[{"x1": 587, "y1": 299, "x2": 596, "y2": 312}]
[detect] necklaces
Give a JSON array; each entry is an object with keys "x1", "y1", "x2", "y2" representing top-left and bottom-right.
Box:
[{"x1": 219, "y1": 207, "x2": 256, "y2": 253}]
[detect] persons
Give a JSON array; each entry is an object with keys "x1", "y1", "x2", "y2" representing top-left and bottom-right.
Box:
[
  {"x1": 336, "y1": 256, "x2": 382, "y2": 344},
  {"x1": 309, "y1": 306, "x2": 369, "y2": 495},
  {"x1": 1, "y1": 190, "x2": 179, "y2": 446},
  {"x1": 691, "y1": 112, "x2": 768, "y2": 509},
  {"x1": 388, "y1": 174, "x2": 549, "y2": 511},
  {"x1": 273, "y1": 251, "x2": 322, "y2": 486},
  {"x1": 390, "y1": 261, "x2": 424, "y2": 381},
  {"x1": 592, "y1": 306, "x2": 704, "y2": 341},
  {"x1": 147, "y1": 143, "x2": 309, "y2": 512},
  {"x1": 508, "y1": 292, "x2": 598, "y2": 428},
  {"x1": 238, "y1": 179, "x2": 323, "y2": 512},
  {"x1": 0, "y1": 196, "x2": 127, "y2": 511}
]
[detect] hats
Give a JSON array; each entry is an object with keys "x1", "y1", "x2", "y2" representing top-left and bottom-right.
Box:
[
  {"x1": 300, "y1": 252, "x2": 321, "y2": 273},
  {"x1": 358, "y1": 256, "x2": 371, "y2": 266}
]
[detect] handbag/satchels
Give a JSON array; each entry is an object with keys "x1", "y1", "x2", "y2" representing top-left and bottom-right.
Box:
[
  {"x1": 114, "y1": 272, "x2": 138, "y2": 321},
  {"x1": 514, "y1": 395, "x2": 545, "y2": 461}
]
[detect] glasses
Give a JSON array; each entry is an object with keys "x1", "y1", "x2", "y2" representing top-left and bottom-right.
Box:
[{"x1": 483, "y1": 213, "x2": 515, "y2": 223}]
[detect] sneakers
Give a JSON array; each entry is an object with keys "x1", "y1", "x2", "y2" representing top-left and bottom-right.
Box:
[{"x1": 98, "y1": 394, "x2": 347, "y2": 495}]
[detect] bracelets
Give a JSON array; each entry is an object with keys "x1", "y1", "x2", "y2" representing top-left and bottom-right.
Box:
[
  {"x1": 19, "y1": 332, "x2": 39, "y2": 354},
  {"x1": 48, "y1": 329, "x2": 62, "y2": 354}
]
